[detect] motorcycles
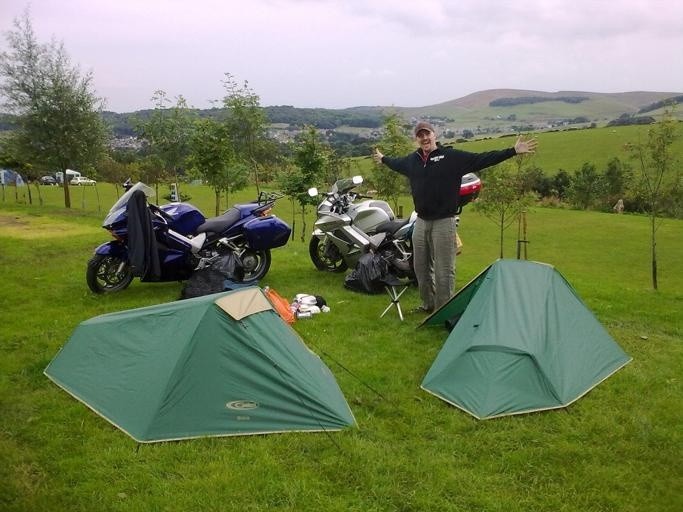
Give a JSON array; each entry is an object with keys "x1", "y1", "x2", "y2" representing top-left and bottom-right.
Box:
[
  {"x1": 308, "y1": 173, "x2": 481, "y2": 286},
  {"x1": 86, "y1": 178, "x2": 291, "y2": 293}
]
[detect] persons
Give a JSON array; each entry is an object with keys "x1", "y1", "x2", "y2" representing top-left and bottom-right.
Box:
[{"x1": 373, "y1": 122, "x2": 537, "y2": 312}]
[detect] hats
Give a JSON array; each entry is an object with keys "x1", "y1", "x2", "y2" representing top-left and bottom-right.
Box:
[{"x1": 413, "y1": 121, "x2": 433, "y2": 137}]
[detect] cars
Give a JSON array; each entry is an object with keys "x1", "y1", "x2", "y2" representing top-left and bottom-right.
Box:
[
  {"x1": 70, "y1": 177, "x2": 96, "y2": 186},
  {"x1": 40, "y1": 176, "x2": 56, "y2": 186}
]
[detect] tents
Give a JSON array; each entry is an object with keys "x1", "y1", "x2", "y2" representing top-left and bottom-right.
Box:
[
  {"x1": 413, "y1": 260, "x2": 633, "y2": 422},
  {"x1": 43, "y1": 286, "x2": 360, "y2": 443}
]
